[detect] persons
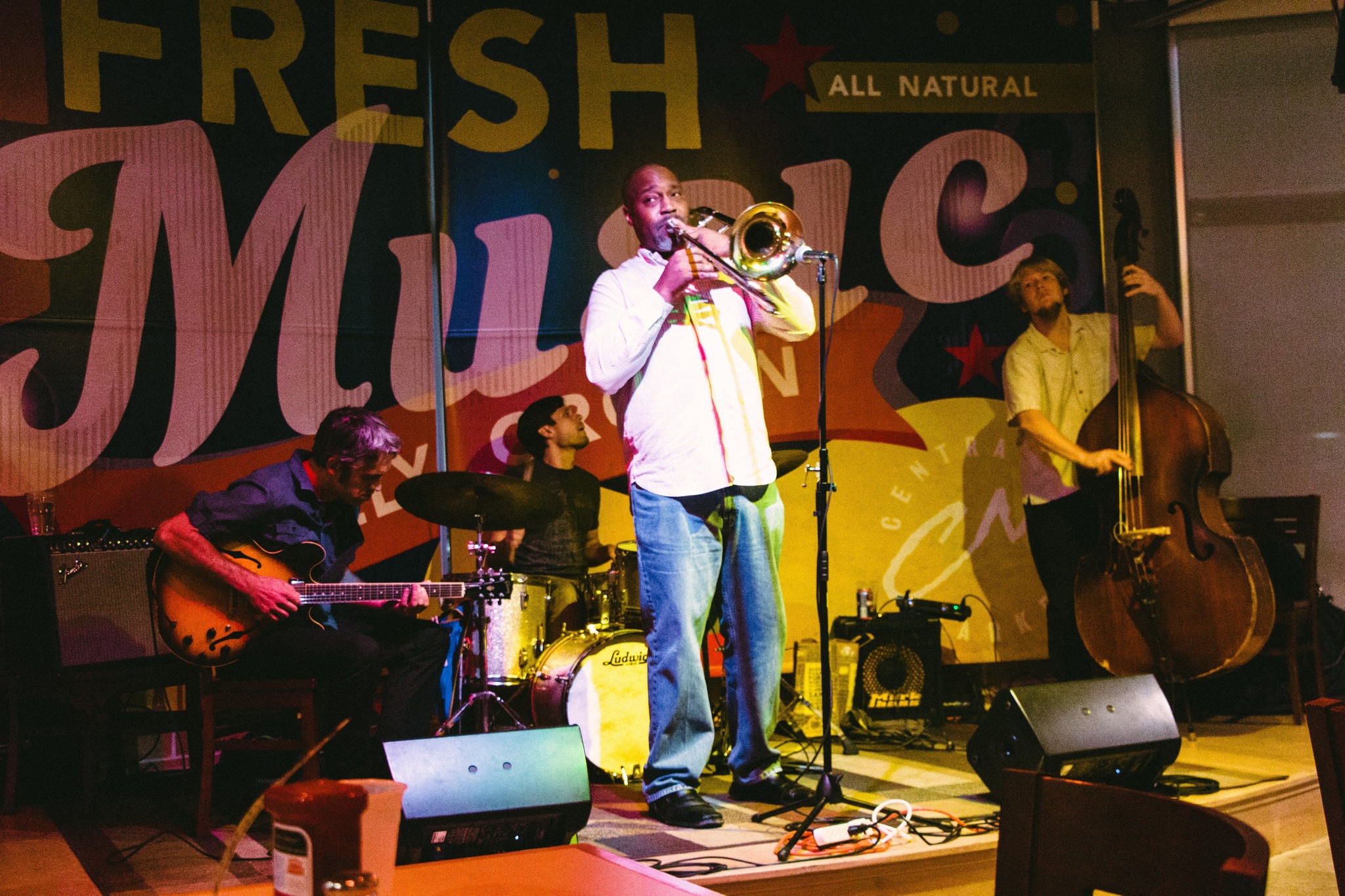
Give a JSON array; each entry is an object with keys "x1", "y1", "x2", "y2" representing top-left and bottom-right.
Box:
[
  {"x1": 149, "y1": 403, "x2": 452, "y2": 788},
  {"x1": 998, "y1": 251, "x2": 1188, "y2": 683},
  {"x1": 478, "y1": 393, "x2": 622, "y2": 649},
  {"x1": 581, "y1": 159, "x2": 823, "y2": 832}
]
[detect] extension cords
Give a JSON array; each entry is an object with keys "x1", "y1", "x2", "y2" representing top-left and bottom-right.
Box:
[{"x1": 895, "y1": 595, "x2": 971, "y2": 622}]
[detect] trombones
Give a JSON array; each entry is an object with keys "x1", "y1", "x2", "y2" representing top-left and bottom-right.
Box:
[{"x1": 664, "y1": 199, "x2": 807, "y2": 316}]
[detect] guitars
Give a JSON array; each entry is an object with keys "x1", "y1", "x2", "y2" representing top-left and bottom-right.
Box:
[{"x1": 143, "y1": 532, "x2": 512, "y2": 670}]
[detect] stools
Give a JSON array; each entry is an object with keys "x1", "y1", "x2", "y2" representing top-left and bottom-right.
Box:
[{"x1": 191, "y1": 661, "x2": 316, "y2": 846}]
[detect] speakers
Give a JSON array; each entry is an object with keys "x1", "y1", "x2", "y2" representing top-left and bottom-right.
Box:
[
  {"x1": 967, "y1": 671, "x2": 1181, "y2": 809},
  {"x1": 372, "y1": 723, "x2": 591, "y2": 867},
  {"x1": 2, "y1": 524, "x2": 183, "y2": 672},
  {"x1": 856, "y1": 619, "x2": 943, "y2": 722},
  {"x1": 994, "y1": 765, "x2": 1269, "y2": 896}
]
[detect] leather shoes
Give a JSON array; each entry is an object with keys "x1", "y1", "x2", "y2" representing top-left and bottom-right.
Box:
[
  {"x1": 730, "y1": 772, "x2": 819, "y2": 806},
  {"x1": 648, "y1": 789, "x2": 726, "y2": 829}
]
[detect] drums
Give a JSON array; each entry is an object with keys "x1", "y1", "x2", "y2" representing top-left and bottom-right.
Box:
[
  {"x1": 533, "y1": 622, "x2": 653, "y2": 784},
  {"x1": 614, "y1": 539, "x2": 646, "y2": 632},
  {"x1": 443, "y1": 568, "x2": 549, "y2": 688}
]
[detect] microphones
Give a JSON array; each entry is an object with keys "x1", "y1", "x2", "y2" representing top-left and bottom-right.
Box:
[{"x1": 795, "y1": 245, "x2": 837, "y2": 265}]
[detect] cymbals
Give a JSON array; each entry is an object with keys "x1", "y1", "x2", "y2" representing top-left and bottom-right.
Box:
[{"x1": 392, "y1": 470, "x2": 565, "y2": 534}]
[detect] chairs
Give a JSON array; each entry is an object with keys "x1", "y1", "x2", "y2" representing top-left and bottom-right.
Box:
[
  {"x1": 1188, "y1": 492, "x2": 1323, "y2": 725},
  {"x1": 991, "y1": 771, "x2": 1268, "y2": 896}
]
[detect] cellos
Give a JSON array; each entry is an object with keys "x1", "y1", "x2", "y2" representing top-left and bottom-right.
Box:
[{"x1": 1069, "y1": 188, "x2": 1278, "y2": 688}]
